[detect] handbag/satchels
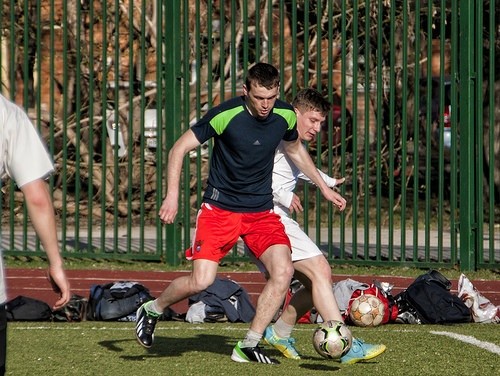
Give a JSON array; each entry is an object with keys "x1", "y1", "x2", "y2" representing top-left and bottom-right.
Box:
[
  {"x1": 52, "y1": 293, "x2": 89, "y2": 321},
  {"x1": 458, "y1": 272, "x2": 498, "y2": 323},
  {"x1": 87, "y1": 281, "x2": 152, "y2": 321}
]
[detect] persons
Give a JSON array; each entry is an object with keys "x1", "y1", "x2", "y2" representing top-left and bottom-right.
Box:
[
  {"x1": 244, "y1": 87, "x2": 387, "y2": 366},
  {"x1": 0, "y1": 92, "x2": 70, "y2": 376},
  {"x1": 135, "y1": 63, "x2": 347, "y2": 366}
]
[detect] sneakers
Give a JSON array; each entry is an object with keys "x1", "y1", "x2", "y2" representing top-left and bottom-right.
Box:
[
  {"x1": 340, "y1": 338, "x2": 387, "y2": 365},
  {"x1": 262, "y1": 326, "x2": 300, "y2": 360},
  {"x1": 231, "y1": 341, "x2": 281, "y2": 365},
  {"x1": 134, "y1": 298, "x2": 163, "y2": 347}
]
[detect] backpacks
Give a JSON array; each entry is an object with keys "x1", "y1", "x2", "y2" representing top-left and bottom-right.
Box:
[{"x1": 403, "y1": 270, "x2": 473, "y2": 324}]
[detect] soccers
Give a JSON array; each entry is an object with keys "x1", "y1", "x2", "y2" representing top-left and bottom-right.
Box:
[
  {"x1": 350, "y1": 294, "x2": 384, "y2": 326},
  {"x1": 311, "y1": 320, "x2": 353, "y2": 359}
]
